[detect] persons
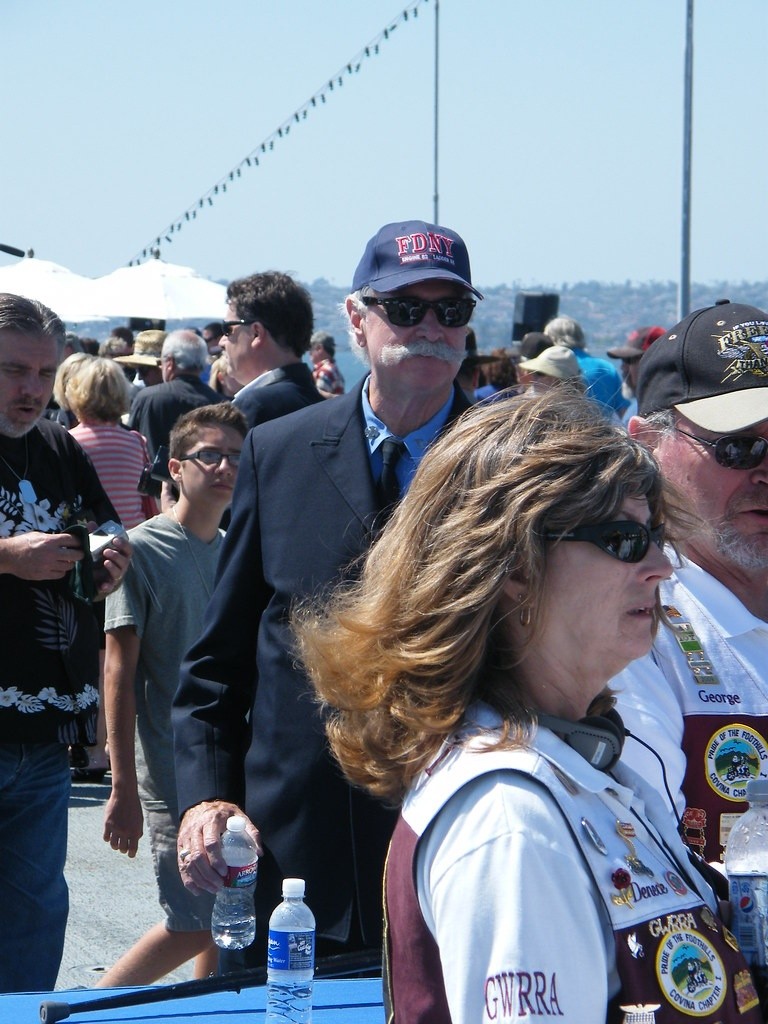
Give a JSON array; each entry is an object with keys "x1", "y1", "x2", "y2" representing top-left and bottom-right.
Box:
[
  {"x1": 0, "y1": 220, "x2": 768, "y2": 1024},
  {"x1": 611, "y1": 296, "x2": 768, "y2": 988},
  {"x1": 172, "y1": 220, "x2": 483, "y2": 963},
  {"x1": 294, "y1": 387, "x2": 768, "y2": 1024}
]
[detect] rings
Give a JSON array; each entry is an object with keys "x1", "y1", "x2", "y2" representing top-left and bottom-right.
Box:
[{"x1": 179, "y1": 848, "x2": 191, "y2": 861}]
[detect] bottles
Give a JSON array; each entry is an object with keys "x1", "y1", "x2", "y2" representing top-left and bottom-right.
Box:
[
  {"x1": 264, "y1": 879, "x2": 316, "y2": 1024},
  {"x1": 726, "y1": 780, "x2": 768, "y2": 976},
  {"x1": 211, "y1": 816, "x2": 259, "y2": 950}
]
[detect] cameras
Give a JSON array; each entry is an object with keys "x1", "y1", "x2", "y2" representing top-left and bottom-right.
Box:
[
  {"x1": 137, "y1": 462, "x2": 180, "y2": 503},
  {"x1": 88, "y1": 519, "x2": 129, "y2": 562}
]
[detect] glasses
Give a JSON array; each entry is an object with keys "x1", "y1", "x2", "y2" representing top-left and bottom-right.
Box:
[
  {"x1": 177, "y1": 450, "x2": 240, "y2": 467},
  {"x1": 360, "y1": 295, "x2": 477, "y2": 329},
  {"x1": 220, "y1": 319, "x2": 255, "y2": 337},
  {"x1": 539, "y1": 517, "x2": 667, "y2": 564},
  {"x1": 651, "y1": 419, "x2": 768, "y2": 471}
]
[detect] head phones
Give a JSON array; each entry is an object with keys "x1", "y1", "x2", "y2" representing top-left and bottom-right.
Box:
[{"x1": 528, "y1": 696, "x2": 625, "y2": 772}]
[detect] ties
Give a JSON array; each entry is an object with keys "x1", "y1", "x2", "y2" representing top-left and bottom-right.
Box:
[{"x1": 376, "y1": 437, "x2": 405, "y2": 517}]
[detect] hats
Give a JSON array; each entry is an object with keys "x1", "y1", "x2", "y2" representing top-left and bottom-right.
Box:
[
  {"x1": 518, "y1": 346, "x2": 580, "y2": 381},
  {"x1": 513, "y1": 332, "x2": 552, "y2": 359},
  {"x1": 306, "y1": 331, "x2": 335, "y2": 351},
  {"x1": 463, "y1": 322, "x2": 501, "y2": 367},
  {"x1": 349, "y1": 221, "x2": 485, "y2": 301},
  {"x1": 635, "y1": 298, "x2": 768, "y2": 433},
  {"x1": 605, "y1": 325, "x2": 670, "y2": 367},
  {"x1": 112, "y1": 330, "x2": 168, "y2": 366}
]
